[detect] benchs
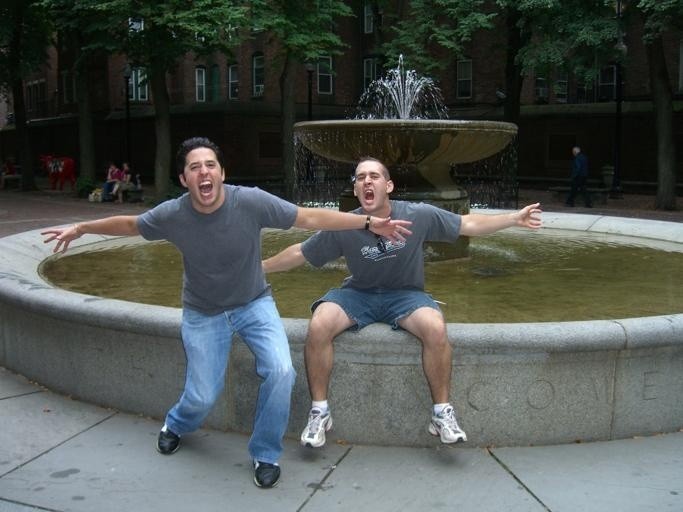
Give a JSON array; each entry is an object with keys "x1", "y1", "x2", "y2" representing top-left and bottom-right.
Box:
[
  {"x1": 548, "y1": 186, "x2": 611, "y2": 208},
  {"x1": 123, "y1": 189, "x2": 144, "y2": 203}
]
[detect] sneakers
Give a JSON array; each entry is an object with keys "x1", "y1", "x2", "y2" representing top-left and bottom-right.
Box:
[
  {"x1": 428, "y1": 406, "x2": 467, "y2": 444},
  {"x1": 156, "y1": 422, "x2": 181, "y2": 454},
  {"x1": 301, "y1": 404, "x2": 332, "y2": 448},
  {"x1": 253, "y1": 457, "x2": 281, "y2": 488}
]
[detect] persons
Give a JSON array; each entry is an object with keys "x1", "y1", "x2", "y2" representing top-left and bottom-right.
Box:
[
  {"x1": 106, "y1": 161, "x2": 137, "y2": 204},
  {"x1": 99, "y1": 164, "x2": 121, "y2": 202},
  {"x1": 562, "y1": 146, "x2": 594, "y2": 209},
  {"x1": 38, "y1": 134, "x2": 414, "y2": 489},
  {"x1": 0, "y1": 159, "x2": 16, "y2": 189},
  {"x1": 260, "y1": 155, "x2": 543, "y2": 450}
]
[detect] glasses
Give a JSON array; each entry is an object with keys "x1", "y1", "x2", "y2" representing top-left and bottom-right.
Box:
[{"x1": 375, "y1": 232, "x2": 386, "y2": 254}]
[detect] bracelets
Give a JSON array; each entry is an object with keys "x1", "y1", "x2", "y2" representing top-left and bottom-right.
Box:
[{"x1": 364, "y1": 215, "x2": 371, "y2": 231}]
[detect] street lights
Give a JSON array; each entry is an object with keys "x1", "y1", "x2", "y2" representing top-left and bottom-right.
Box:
[
  {"x1": 612, "y1": 42, "x2": 626, "y2": 187},
  {"x1": 305, "y1": 60, "x2": 315, "y2": 121},
  {"x1": 122, "y1": 62, "x2": 132, "y2": 172}
]
